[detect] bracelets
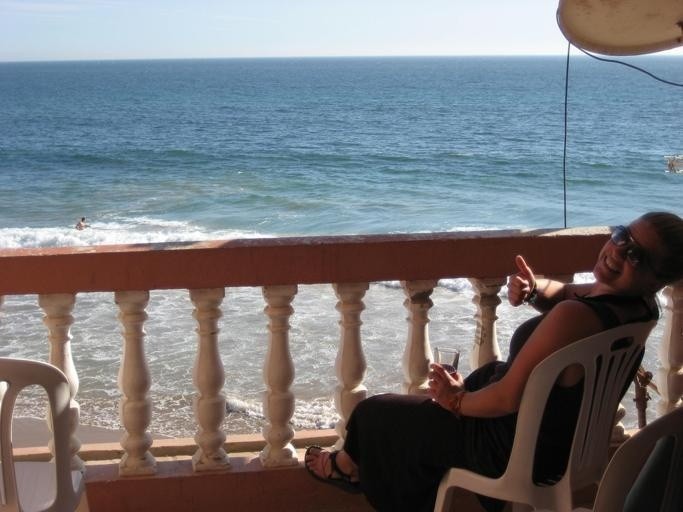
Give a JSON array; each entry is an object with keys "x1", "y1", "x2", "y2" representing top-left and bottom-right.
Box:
[
  {"x1": 528, "y1": 280, "x2": 542, "y2": 306},
  {"x1": 449, "y1": 389, "x2": 469, "y2": 412}
]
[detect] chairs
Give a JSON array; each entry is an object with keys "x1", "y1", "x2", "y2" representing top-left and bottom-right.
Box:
[{"x1": 0, "y1": 358, "x2": 86, "y2": 511}]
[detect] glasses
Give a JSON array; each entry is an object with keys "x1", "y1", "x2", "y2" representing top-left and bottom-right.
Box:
[{"x1": 609, "y1": 223, "x2": 654, "y2": 272}]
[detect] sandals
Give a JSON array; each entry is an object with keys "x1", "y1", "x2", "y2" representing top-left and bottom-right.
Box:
[{"x1": 304, "y1": 442, "x2": 364, "y2": 496}]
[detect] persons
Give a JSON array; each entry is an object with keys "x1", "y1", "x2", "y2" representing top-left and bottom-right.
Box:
[
  {"x1": 305, "y1": 208, "x2": 683, "y2": 512},
  {"x1": 75, "y1": 216, "x2": 91, "y2": 230}
]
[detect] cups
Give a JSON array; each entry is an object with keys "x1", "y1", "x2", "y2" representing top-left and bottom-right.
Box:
[{"x1": 434, "y1": 346, "x2": 460, "y2": 382}]
